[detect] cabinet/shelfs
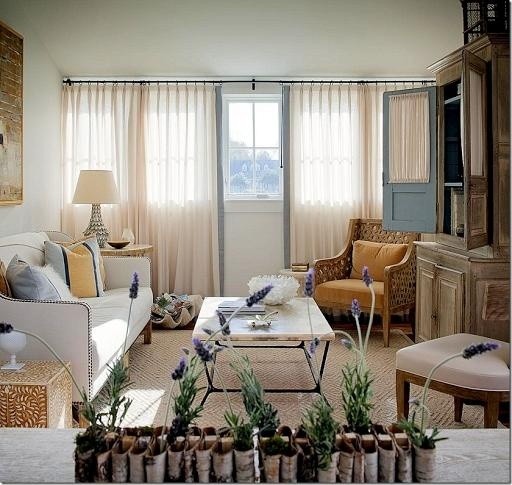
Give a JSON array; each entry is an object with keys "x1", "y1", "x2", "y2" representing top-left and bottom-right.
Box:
[
  {"x1": 383, "y1": 38, "x2": 510, "y2": 258},
  {"x1": 413, "y1": 240, "x2": 510, "y2": 345}
]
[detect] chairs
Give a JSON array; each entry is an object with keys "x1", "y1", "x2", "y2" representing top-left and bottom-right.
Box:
[{"x1": 313, "y1": 218, "x2": 421, "y2": 348}]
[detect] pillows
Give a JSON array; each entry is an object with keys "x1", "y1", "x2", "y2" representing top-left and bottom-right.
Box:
[
  {"x1": 43, "y1": 237, "x2": 105, "y2": 299},
  {"x1": 6, "y1": 253, "x2": 79, "y2": 302},
  {"x1": 0, "y1": 259, "x2": 12, "y2": 297}
]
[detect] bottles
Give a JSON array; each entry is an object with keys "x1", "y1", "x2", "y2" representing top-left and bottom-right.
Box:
[{"x1": 122, "y1": 228, "x2": 134, "y2": 245}]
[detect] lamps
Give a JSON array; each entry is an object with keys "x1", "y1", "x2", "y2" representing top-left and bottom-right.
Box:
[{"x1": 72, "y1": 170, "x2": 119, "y2": 248}]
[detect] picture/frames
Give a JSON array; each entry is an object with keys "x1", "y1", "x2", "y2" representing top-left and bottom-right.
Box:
[{"x1": 0, "y1": 18, "x2": 24, "y2": 207}]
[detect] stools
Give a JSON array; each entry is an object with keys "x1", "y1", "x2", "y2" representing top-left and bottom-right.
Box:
[{"x1": 395, "y1": 332, "x2": 510, "y2": 429}]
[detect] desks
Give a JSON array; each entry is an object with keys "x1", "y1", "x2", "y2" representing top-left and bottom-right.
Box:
[
  {"x1": 99, "y1": 243, "x2": 153, "y2": 256},
  {"x1": 192, "y1": 297, "x2": 336, "y2": 408},
  {"x1": 0, "y1": 361, "x2": 73, "y2": 429},
  {"x1": 1, "y1": 428, "x2": 509, "y2": 485}
]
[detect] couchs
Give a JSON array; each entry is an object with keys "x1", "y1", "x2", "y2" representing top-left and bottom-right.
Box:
[{"x1": 0, "y1": 233, "x2": 153, "y2": 406}]
